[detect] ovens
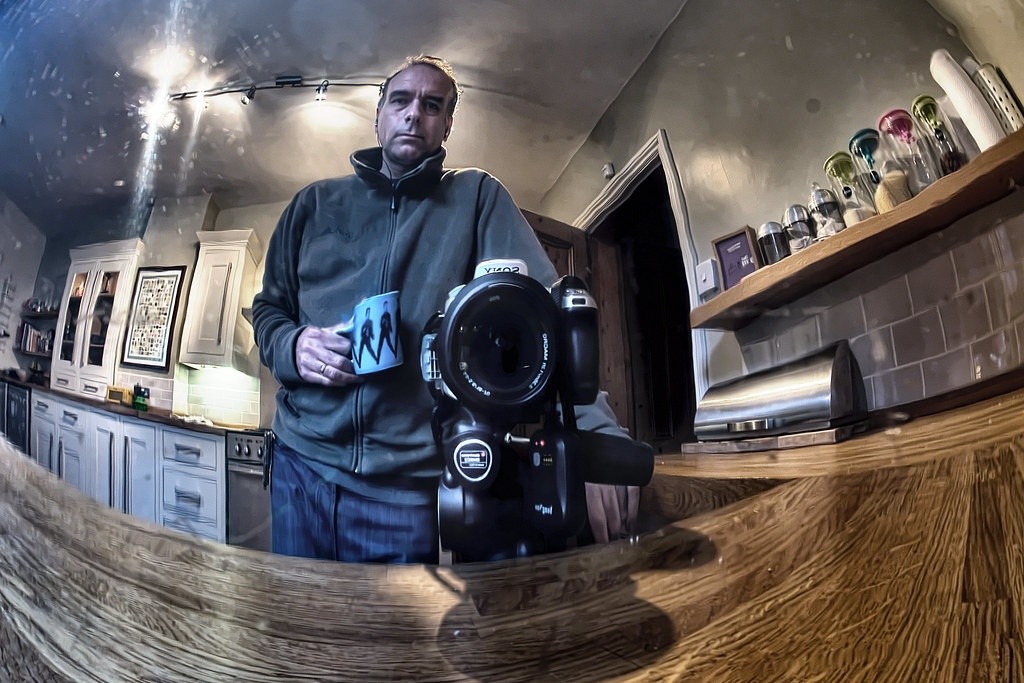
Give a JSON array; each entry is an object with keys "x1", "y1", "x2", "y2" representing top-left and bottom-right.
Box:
[{"x1": 226, "y1": 431, "x2": 275, "y2": 552}]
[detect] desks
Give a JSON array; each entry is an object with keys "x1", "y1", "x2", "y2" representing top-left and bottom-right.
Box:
[{"x1": 0, "y1": 433, "x2": 1024, "y2": 683}]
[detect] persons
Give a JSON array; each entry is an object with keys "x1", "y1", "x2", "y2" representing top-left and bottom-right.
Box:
[
  {"x1": 254, "y1": 59, "x2": 642, "y2": 567},
  {"x1": 351, "y1": 298, "x2": 402, "y2": 367}
]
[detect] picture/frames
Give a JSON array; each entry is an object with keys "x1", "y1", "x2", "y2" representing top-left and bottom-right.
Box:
[{"x1": 119, "y1": 265, "x2": 187, "y2": 374}]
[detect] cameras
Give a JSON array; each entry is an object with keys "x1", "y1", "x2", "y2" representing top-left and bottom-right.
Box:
[{"x1": 420, "y1": 259, "x2": 599, "y2": 424}]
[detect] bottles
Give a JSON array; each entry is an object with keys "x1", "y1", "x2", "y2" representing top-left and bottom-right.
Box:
[{"x1": 755, "y1": 95, "x2": 967, "y2": 266}]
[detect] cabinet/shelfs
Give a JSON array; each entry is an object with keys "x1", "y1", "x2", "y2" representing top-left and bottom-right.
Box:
[
  {"x1": 49, "y1": 237, "x2": 143, "y2": 401},
  {"x1": 16, "y1": 309, "x2": 59, "y2": 359},
  {"x1": 88, "y1": 406, "x2": 160, "y2": 526},
  {"x1": 29, "y1": 388, "x2": 87, "y2": 495},
  {"x1": 159, "y1": 423, "x2": 227, "y2": 542}
]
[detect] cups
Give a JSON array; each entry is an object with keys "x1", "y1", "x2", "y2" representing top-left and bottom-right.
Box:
[
  {"x1": 332, "y1": 291, "x2": 403, "y2": 376},
  {"x1": 133, "y1": 386, "x2": 150, "y2": 411}
]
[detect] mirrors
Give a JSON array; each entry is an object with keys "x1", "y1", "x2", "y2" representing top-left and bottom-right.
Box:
[{"x1": 0, "y1": 0, "x2": 1024, "y2": 683}]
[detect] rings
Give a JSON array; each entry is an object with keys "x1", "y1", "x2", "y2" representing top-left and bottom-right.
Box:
[{"x1": 319, "y1": 363, "x2": 328, "y2": 376}]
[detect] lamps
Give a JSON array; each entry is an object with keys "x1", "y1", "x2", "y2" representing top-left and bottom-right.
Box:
[
  {"x1": 316, "y1": 80, "x2": 330, "y2": 102},
  {"x1": 240, "y1": 85, "x2": 257, "y2": 106}
]
